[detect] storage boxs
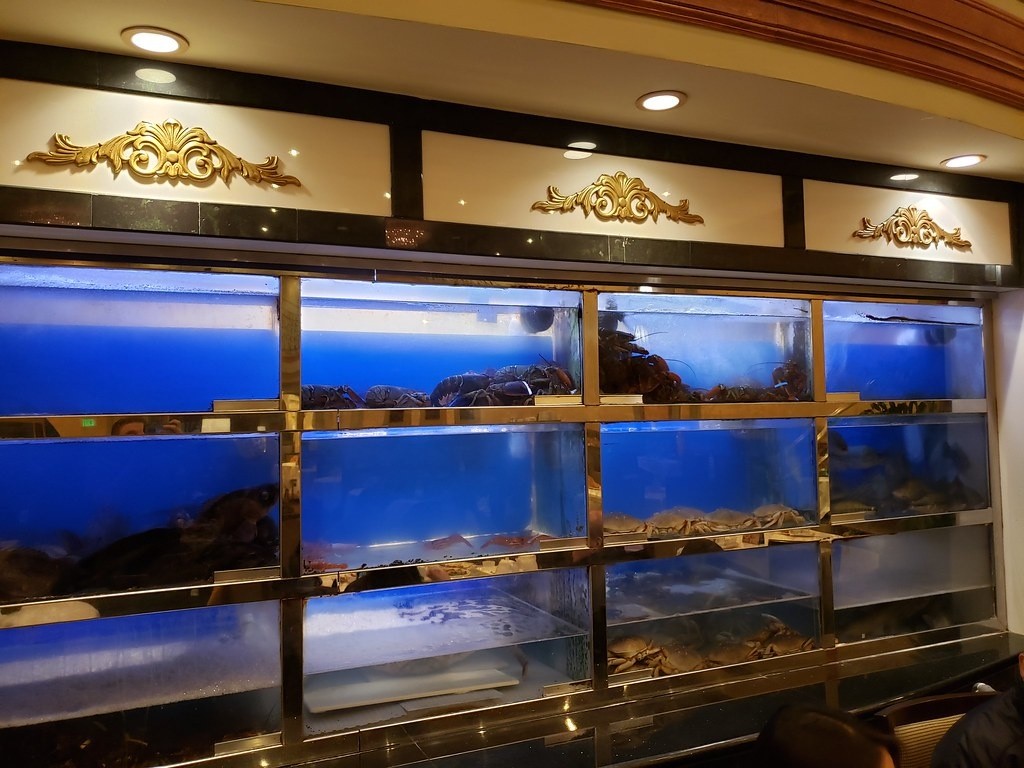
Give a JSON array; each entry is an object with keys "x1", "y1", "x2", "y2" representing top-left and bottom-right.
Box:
[{"x1": 1, "y1": 255, "x2": 1008, "y2": 768}]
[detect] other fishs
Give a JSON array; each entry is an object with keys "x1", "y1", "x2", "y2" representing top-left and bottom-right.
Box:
[
  {"x1": 827, "y1": 428, "x2": 989, "y2": 515},
  {"x1": 1, "y1": 478, "x2": 281, "y2": 603}
]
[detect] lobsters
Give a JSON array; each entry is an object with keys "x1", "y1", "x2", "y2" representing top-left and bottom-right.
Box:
[
  {"x1": 301, "y1": 352, "x2": 582, "y2": 407},
  {"x1": 594, "y1": 310, "x2": 814, "y2": 402}
]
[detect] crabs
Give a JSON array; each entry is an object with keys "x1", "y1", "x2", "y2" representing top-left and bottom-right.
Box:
[
  {"x1": 601, "y1": 501, "x2": 811, "y2": 540},
  {"x1": 606, "y1": 610, "x2": 817, "y2": 678}
]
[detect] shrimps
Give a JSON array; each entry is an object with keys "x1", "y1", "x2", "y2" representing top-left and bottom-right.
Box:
[{"x1": 301, "y1": 531, "x2": 560, "y2": 574}]
